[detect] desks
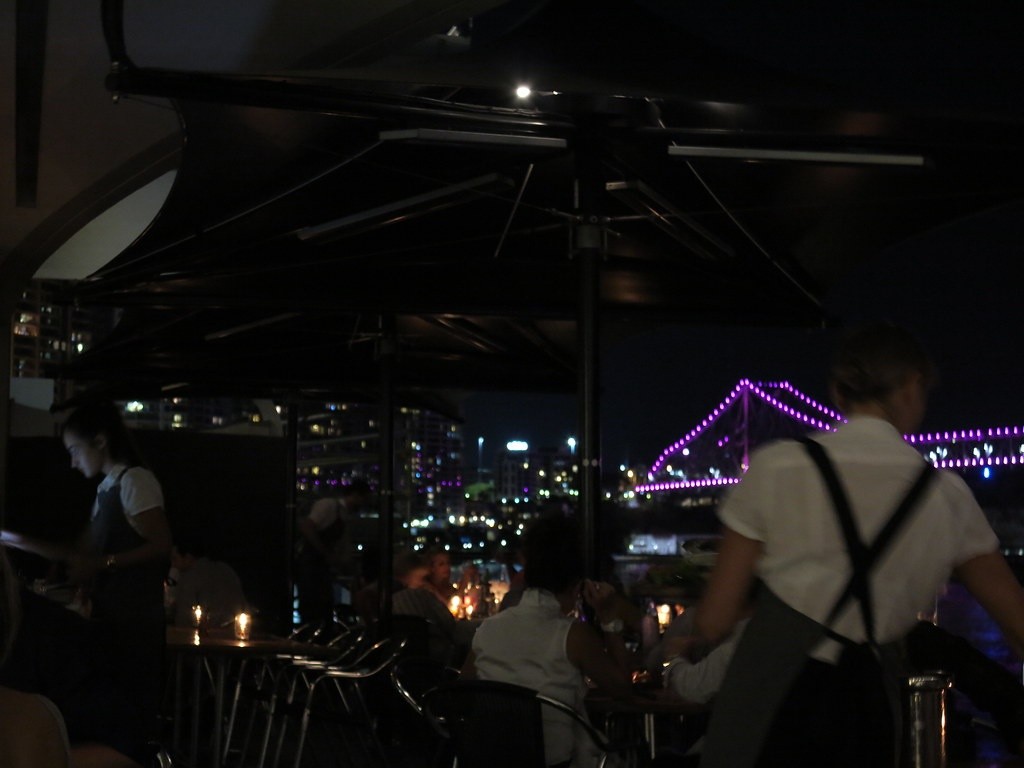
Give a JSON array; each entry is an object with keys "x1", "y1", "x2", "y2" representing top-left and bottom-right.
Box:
[{"x1": 165, "y1": 624, "x2": 342, "y2": 768}]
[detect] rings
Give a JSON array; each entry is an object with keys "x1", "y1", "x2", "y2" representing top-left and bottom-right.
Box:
[{"x1": 595, "y1": 585, "x2": 601, "y2": 591}]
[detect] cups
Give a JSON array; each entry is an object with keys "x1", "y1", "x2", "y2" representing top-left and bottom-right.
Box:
[
  {"x1": 191, "y1": 602, "x2": 206, "y2": 628},
  {"x1": 233, "y1": 608, "x2": 251, "y2": 640}
]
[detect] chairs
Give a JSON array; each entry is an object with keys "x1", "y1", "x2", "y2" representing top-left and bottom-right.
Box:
[{"x1": 203, "y1": 598, "x2": 641, "y2": 768}]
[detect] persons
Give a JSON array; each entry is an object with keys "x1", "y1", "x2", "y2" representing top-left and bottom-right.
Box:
[
  {"x1": 0, "y1": 398, "x2": 657, "y2": 768},
  {"x1": 661, "y1": 316, "x2": 1024, "y2": 768}
]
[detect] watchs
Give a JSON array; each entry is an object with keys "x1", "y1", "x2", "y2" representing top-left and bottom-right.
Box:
[
  {"x1": 106, "y1": 553, "x2": 116, "y2": 569},
  {"x1": 600, "y1": 619, "x2": 624, "y2": 632}
]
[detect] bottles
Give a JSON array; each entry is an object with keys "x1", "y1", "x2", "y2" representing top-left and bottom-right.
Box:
[{"x1": 574, "y1": 592, "x2": 586, "y2": 623}]
